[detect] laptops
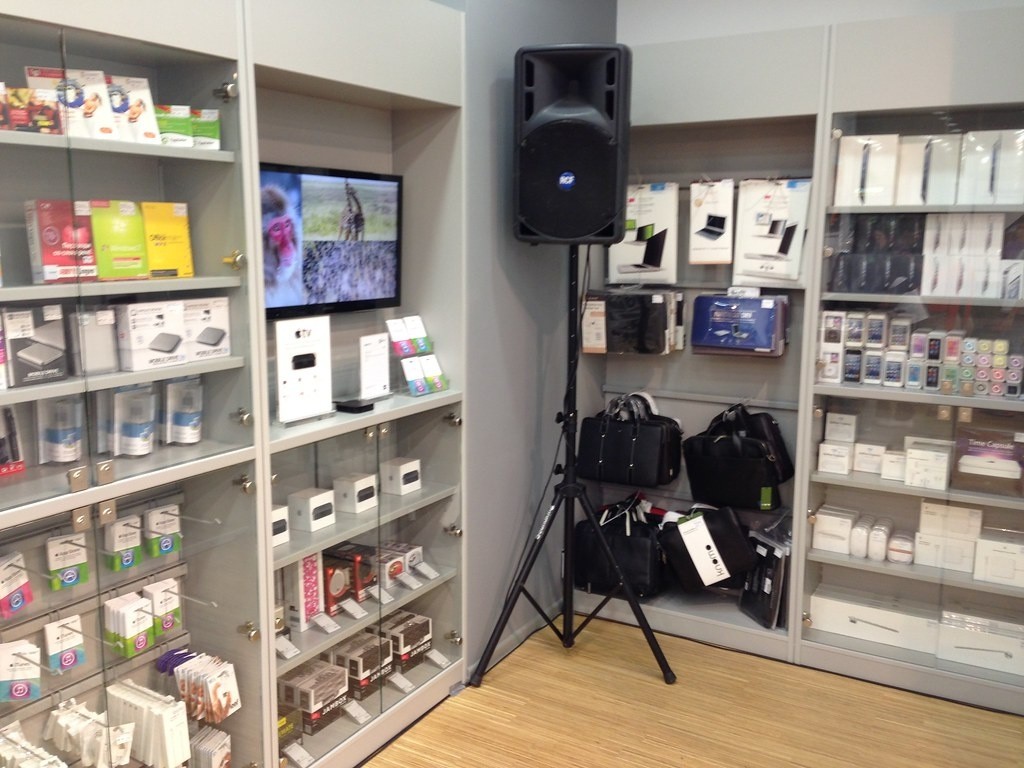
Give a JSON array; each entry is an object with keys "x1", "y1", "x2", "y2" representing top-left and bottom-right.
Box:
[
  {"x1": 695, "y1": 215, "x2": 726, "y2": 240},
  {"x1": 740, "y1": 220, "x2": 809, "y2": 281},
  {"x1": 617, "y1": 219, "x2": 668, "y2": 273}
]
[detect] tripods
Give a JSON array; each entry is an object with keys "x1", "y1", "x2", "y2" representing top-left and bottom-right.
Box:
[{"x1": 470, "y1": 244, "x2": 676, "y2": 688}]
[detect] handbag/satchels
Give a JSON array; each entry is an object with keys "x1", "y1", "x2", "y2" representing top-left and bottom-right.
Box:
[
  {"x1": 579, "y1": 394, "x2": 683, "y2": 488},
  {"x1": 654, "y1": 502, "x2": 756, "y2": 588},
  {"x1": 574, "y1": 495, "x2": 678, "y2": 604},
  {"x1": 682, "y1": 403, "x2": 793, "y2": 511}
]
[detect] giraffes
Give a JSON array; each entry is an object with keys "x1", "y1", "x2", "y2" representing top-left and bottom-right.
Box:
[{"x1": 336, "y1": 178, "x2": 365, "y2": 241}]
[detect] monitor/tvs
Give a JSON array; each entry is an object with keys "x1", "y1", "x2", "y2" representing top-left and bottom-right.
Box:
[{"x1": 258, "y1": 162, "x2": 402, "y2": 320}]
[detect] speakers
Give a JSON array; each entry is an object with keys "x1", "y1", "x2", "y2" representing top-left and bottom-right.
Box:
[{"x1": 514, "y1": 43, "x2": 633, "y2": 247}]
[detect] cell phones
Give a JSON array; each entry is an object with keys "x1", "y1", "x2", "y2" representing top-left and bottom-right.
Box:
[{"x1": 820, "y1": 314, "x2": 941, "y2": 389}]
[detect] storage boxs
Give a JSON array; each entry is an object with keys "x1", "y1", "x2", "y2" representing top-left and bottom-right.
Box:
[
  {"x1": 914, "y1": 497, "x2": 1024, "y2": 591},
  {"x1": 811, "y1": 502, "x2": 860, "y2": 556},
  {"x1": 820, "y1": 128, "x2": 1024, "y2": 298},
  {"x1": 273, "y1": 540, "x2": 434, "y2": 758},
  {"x1": 333, "y1": 472, "x2": 380, "y2": 513},
  {"x1": 286, "y1": 486, "x2": 337, "y2": 532},
  {"x1": 379, "y1": 456, "x2": 421, "y2": 495},
  {"x1": 74, "y1": 296, "x2": 230, "y2": 374},
  {"x1": 22, "y1": 199, "x2": 100, "y2": 284},
  {"x1": 271, "y1": 504, "x2": 291, "y2": 545},
  {"x1": 817, "y1": 406, "x2": 957, "y2": 494}
]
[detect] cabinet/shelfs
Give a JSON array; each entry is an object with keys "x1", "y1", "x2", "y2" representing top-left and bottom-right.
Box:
[
  {"x1": 242, "y1": 0, "x2": 471, "y2": 768},
  {"x1": 793, "y1": 11, "x2": 1024, "y2": 717},
  {"x1": 560, "y1": 23, "x2": 835, "y2": 669},
  {"x1": 0, "y1": 0, "x2": 274, "y2": 768}
]
